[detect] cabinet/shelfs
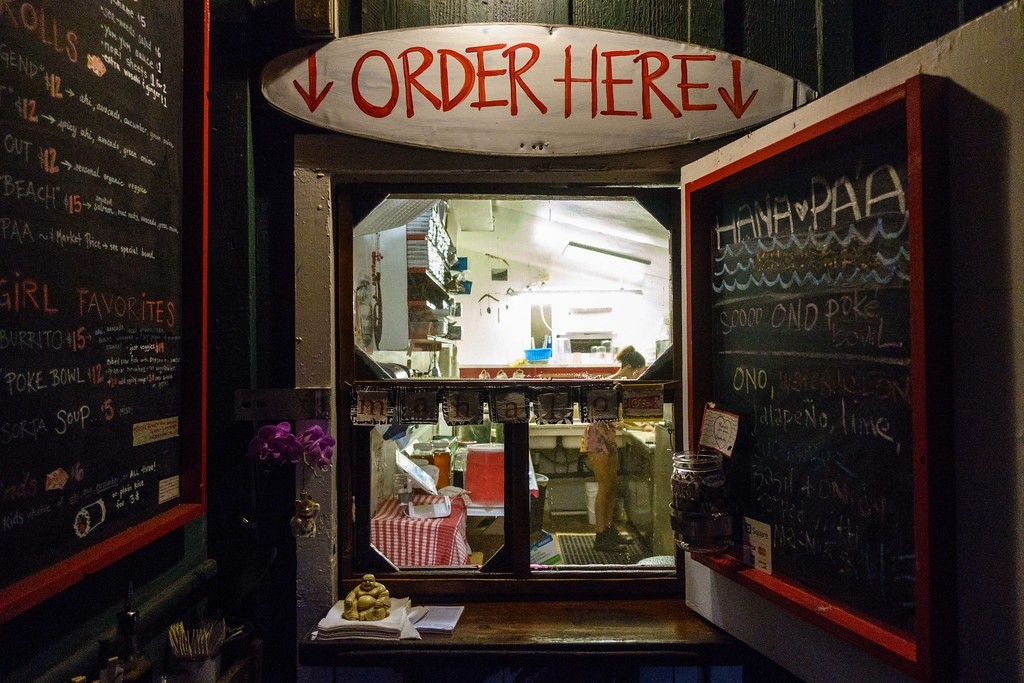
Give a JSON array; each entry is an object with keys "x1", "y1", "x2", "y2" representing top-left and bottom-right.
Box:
[{"x1": 406, "y1": 210, "x2": 455, "y2": 345}]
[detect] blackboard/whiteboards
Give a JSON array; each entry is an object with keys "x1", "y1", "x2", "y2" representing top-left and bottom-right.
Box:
[
  {"x1": 684, "y1": 72, "x2": 950, "y2": 683},
  {"x1": 0, "y1": 0, "x2": 211, "y2": 621}
]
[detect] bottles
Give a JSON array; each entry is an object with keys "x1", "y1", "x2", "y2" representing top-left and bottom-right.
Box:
[
  {"x1": 410, "y1": 443, "x2": 434, "y2": 494},
  {"x1": 671, "y1": 451, "x2": 728, "y2": 553},
  {"x1": 390, "y1": 474, "x2": 408, "y2": 500},
  {"x1": 431, "y1": 440, "x2": 450, "y2": 488},
  {"x1": 454, "y1": 441, "x2": 477, "y2": 503}
]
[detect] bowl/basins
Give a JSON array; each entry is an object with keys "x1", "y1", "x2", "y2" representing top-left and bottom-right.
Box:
[
  {"x1": 524, "y1": 347, "x2": 552, "y2": 362},
  {"x1": 191, "y1": 616, "x2": 255, "y2": 669}
]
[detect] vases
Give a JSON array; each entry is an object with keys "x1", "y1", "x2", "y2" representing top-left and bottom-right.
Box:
[{"x1": 291, "y1": 492, "x2": 320, "y2": 540}]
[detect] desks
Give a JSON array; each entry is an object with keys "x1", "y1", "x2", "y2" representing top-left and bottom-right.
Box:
[
  {"x1": 297, "y1": 600, "x2": 745, "y2": 683},
  {"x1": 371, "y1": 494, "x2": 471, "y2": 566}
]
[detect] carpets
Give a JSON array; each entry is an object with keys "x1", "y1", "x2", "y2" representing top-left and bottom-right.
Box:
[{"x1": 556, "y1": 531, "x2": 655, "y2": 565}]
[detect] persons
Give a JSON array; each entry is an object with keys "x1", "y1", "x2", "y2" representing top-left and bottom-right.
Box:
[
  {"x1": 580, "y1": 345, "x2": 647, "y2": 553},
  {"x1": 342, "y1": 574, "x2": 391, "y2": 621}
]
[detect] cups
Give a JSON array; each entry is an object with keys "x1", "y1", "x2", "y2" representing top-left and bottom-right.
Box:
[
  {"x1": 555, "y1": 338, "x2": 571, "y2": 364},
  {"x1": 590, "y1": 340, "x2": 612, "y2": 364},
  {"x1": 176, "y1": 655, "x2": 221, "y2": 683},
  {"x1": 529, "y1": 472, "x2": 549, "y2": 533}
]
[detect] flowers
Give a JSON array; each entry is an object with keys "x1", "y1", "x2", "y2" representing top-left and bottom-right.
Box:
[{"x1": 246, "y1": 422, "x2": 335, "y2": 491}]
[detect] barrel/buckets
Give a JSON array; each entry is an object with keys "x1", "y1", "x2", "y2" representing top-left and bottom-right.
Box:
[
  {"x1": 586, "y1": 482, "x2": 599, "y2": 525},
  {"x1": 466, "y1": 443, "x2": 505, "y2": 506}
]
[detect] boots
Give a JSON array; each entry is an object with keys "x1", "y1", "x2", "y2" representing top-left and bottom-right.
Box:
[
  {"x1": 605, "y1": 523, "x2": 634, "y2": 544},
  {"x1": 594, "y1": 528, "x2": 627, "y2": 552}
]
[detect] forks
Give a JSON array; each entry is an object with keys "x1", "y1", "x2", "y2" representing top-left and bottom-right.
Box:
[{"x1": 169, "y1": 619, "x2": 226, "y2": 657}]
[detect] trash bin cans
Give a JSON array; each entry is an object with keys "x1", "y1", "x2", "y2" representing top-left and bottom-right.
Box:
[{"x1": 529, "y1": 472, "x2": 550, "y2": 532}]
